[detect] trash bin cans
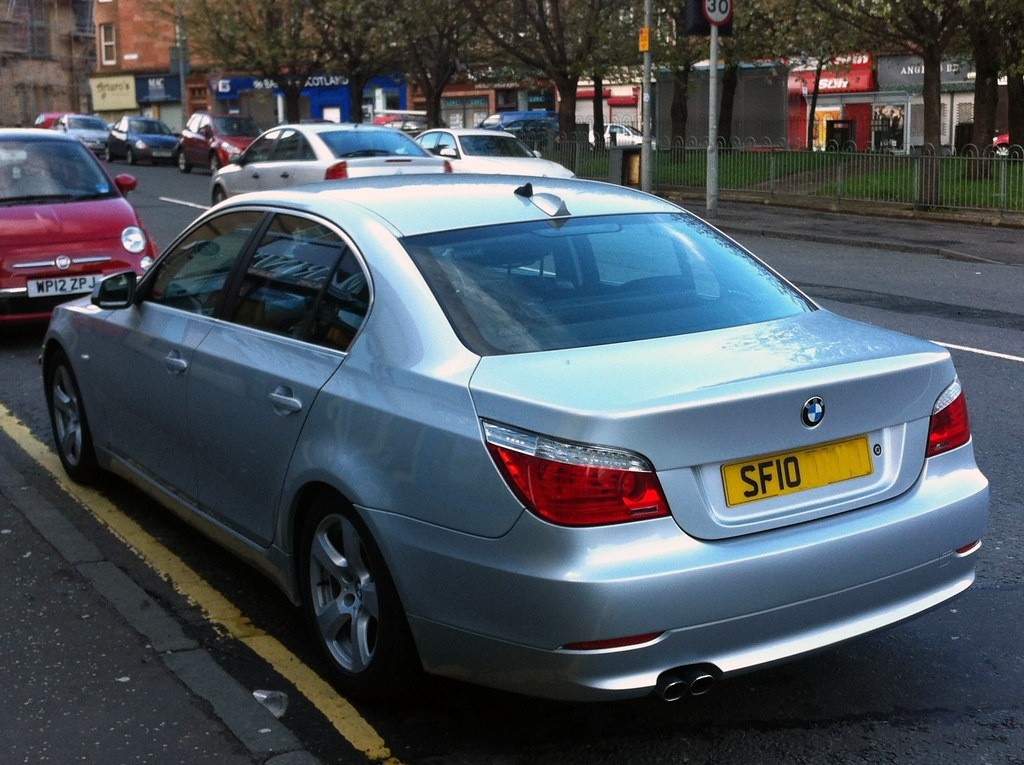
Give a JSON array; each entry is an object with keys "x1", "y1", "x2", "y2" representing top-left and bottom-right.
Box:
[{"x1": 825, "y1": 120, "x2": 856, "y2": 153}]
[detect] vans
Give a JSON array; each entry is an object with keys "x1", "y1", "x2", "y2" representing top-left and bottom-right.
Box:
[{"x1": 479, "y1": 110, "x2": 563, "y2": 152}]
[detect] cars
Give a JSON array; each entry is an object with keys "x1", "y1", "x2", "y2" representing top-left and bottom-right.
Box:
[
  {"x1": 171, "y1": 109, "x2": 270, "y2": 174},
  {"x1": 34, "y1": 110, "x2": 69, "y2": 130},
  {"x1": 38, "y1": 171, "x2": 994, "y2": 721},
  {"x1": 208, "y1": 122, "x2": 455, "y2": 211},
  {"x1": 0, "y1": 125, "x2": 161, "y2": 330},
  {"x1": 105, "y1": 114, "x2": 180, "y2": 166},
  {"x1": 590, "y1": 124, "x2": 656, "y2": 147},
  {"x1": 384, "y1": 118, "x2": 448, "y2": 138},
  {"x1": 412, "y1": 127, "x2": 576, "y2": 181},
  {"x1": 48, "y1": 112, "x2": 115, "y2": 159}
]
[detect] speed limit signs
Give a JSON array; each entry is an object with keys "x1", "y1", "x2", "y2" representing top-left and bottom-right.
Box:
[{"x1": 704, "y1": 0, "x2": 735, "y2": 24}]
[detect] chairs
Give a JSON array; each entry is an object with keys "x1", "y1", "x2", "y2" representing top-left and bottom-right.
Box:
[{"x1": 488, "y1": 239, "x2": 561, "y2": 297}]
